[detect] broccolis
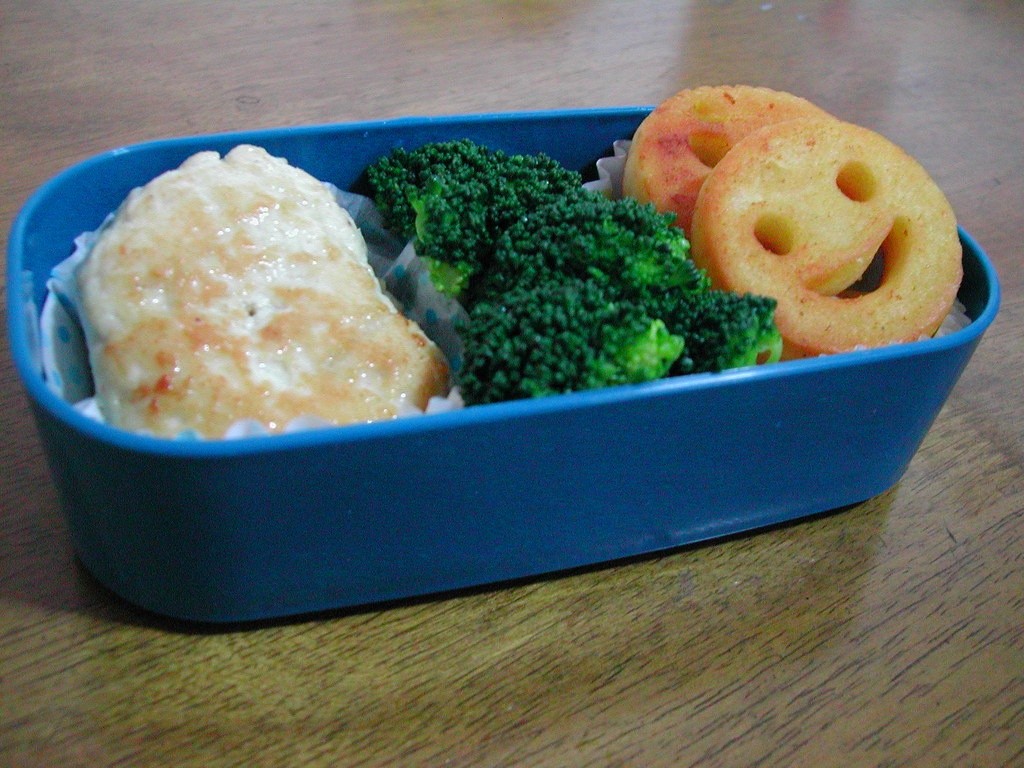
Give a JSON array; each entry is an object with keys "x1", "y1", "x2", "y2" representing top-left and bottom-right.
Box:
[{"x1": 364, "y1": 143, "x2": 784, "y2": 409}]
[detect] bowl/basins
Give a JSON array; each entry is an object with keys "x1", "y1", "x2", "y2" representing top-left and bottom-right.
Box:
[{"x1": 6, "y1": 107, "x2": 1001, "y2": 623}]
